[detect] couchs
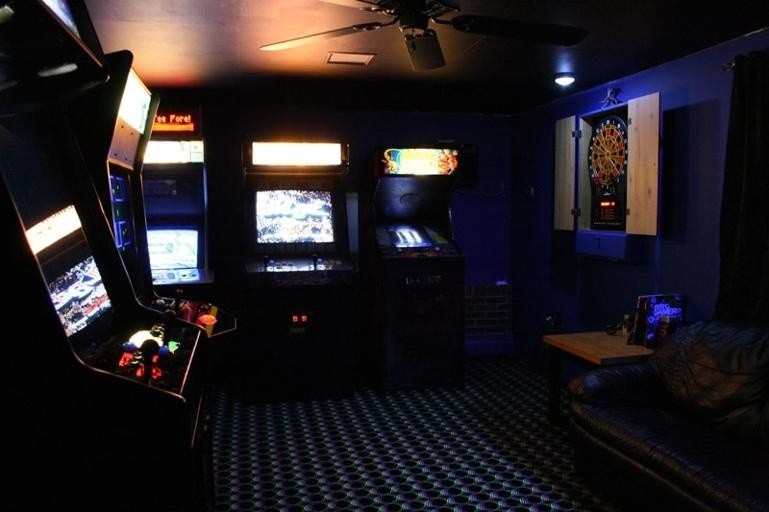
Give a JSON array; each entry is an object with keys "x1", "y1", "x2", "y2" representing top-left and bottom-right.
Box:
[{"x1": 565, "y1": 320, "x2": 768, "y2": 512}]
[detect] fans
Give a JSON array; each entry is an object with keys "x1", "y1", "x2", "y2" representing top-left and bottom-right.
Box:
[{"x1": 254, "y1": 0, "x2": 596, "y2": 74}]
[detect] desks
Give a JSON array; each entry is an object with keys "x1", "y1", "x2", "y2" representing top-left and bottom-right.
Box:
[{"x1": 542, "y1": 327, "x2": 655, "y2": 428}]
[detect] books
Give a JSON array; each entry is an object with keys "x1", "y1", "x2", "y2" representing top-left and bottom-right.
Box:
[{"x1": 632, "y1": 293, "x2": 688, "y2": 349}]
